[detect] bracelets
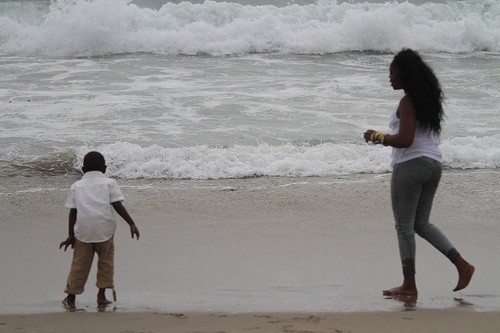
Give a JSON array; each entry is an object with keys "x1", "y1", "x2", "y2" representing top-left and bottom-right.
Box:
[{"x1": 370, "y1": 130, "x2": 390, "y2": 147}]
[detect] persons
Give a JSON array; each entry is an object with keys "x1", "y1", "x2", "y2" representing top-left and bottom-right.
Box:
[
  {"x1": 58, "y1": 150, "x2": 140, "y2": 312},
  {"x1": 364, "y1": 46, "x2": 477, "y2": 297}
]
